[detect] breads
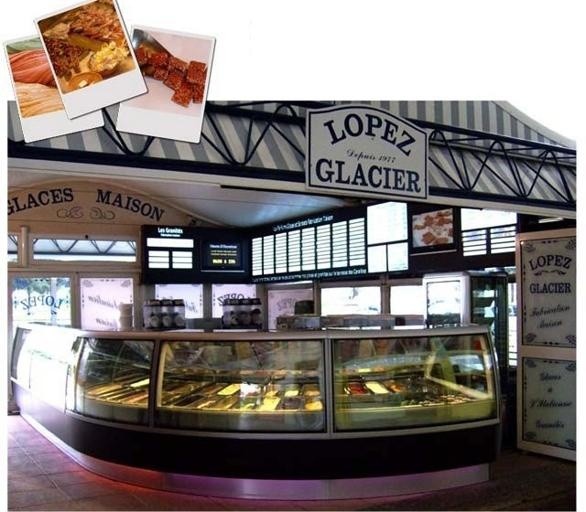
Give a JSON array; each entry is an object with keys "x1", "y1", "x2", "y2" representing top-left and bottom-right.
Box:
[{"x1": 8, "y1": 46, "x2": 65, "y2": 119}]
